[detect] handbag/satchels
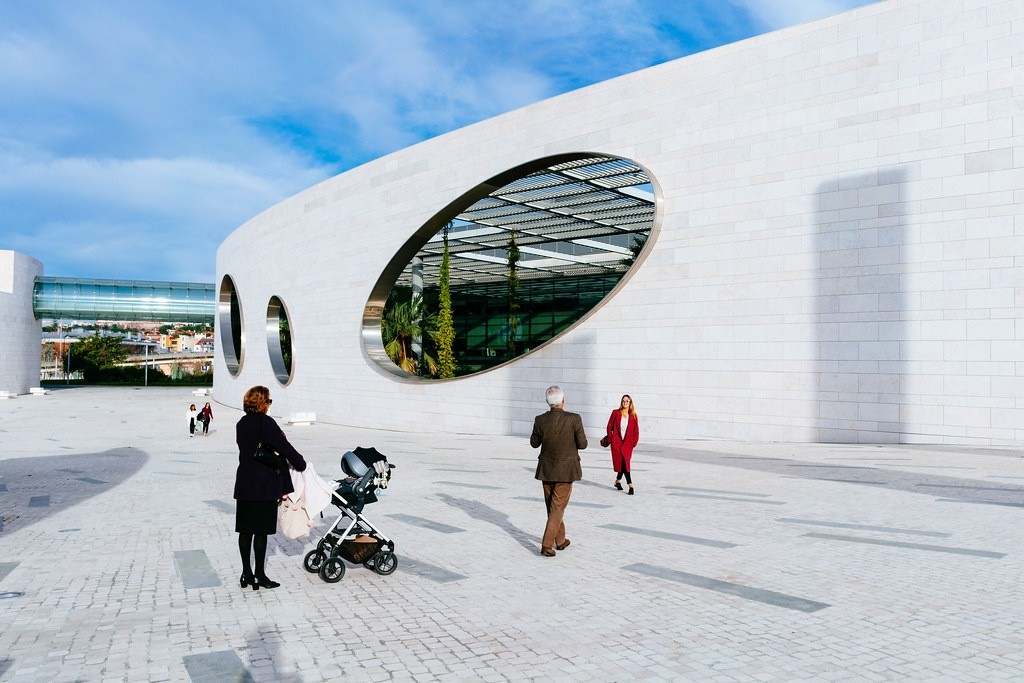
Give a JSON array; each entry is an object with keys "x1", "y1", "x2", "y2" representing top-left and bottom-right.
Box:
[
  {"x1": 252, "y1": 440, "x2": 287, "y2": 475},
  {"x1": 600, "y1": 434, "x2": 611, "y2": 448},
  {"x1": 289, "y1": 461, "x2": 333, "y2": 521},
  {"x1": 276, "y1": 495, "x2": 315, "y2": 541}
]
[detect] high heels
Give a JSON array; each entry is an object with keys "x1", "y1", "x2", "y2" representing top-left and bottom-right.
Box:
[
  {"x1": 240, "y1": 573, "x2": 255, "y2": 588},
  {"x1": 252, "y1": 574, "x2": 281, "y2": 590}
]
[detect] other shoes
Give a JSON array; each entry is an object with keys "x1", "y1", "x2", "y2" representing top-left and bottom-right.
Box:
[
  {"x1": 205, "y1": 432, "x2": 208, "y2": 437},
  {"x1": 202, "y1": 431, "x2": 205, "y2": 436},
  {"x1": 190, "y1": 434, "x2": 194, "y2": 437}
]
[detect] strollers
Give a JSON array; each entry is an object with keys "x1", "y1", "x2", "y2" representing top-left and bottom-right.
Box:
[{"x1": 285, "y1": 446, "x2": 398, "y2": 584}]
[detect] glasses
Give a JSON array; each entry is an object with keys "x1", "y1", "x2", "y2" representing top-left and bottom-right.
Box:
[
  {"x1": 621, "y1": 399, "x2": 631, "y2": 402},
  {"x1": 268, "y1": 399, "x2": 273, "y2": 404}
]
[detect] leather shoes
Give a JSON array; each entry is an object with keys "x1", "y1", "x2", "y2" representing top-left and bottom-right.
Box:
[
  {"x1": 541, "y1": 546, "x2": 556, "y2": 557},
  {"x1": 557, "y1": 539, "x2": 570, "y2": 550}
]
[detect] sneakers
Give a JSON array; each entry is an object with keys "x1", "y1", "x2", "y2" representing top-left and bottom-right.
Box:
[
  {"x1": 627, "y1": 487, "x2": 634, "y2": 495},
  {"x1": 614, "y1": 482, "x2": 623, "y2": 490}
]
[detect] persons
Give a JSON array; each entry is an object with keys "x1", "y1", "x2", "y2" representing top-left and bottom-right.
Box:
[
  {"x1": 530, "y1": 386, "x2": 588, "y2": 557},
  {"x1": 186, "y1": 402, "x2": 214, "y2": 437},
  {"x1": 607, "y1": 395, "x2": 639, "y2": 495},
  {"x1": 233, "y1": 386, "x2": 306, "y2": 591}
]
